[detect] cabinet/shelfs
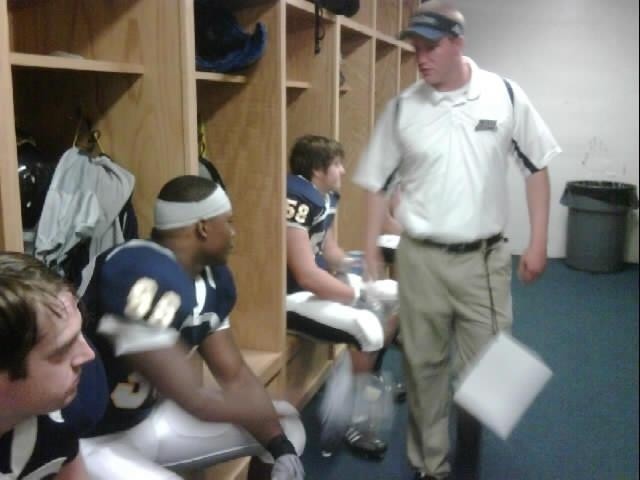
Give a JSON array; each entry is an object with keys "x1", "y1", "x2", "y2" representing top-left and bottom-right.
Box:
[{"x1": 0, "y1": 1, "x2": 424, "y2": 479}]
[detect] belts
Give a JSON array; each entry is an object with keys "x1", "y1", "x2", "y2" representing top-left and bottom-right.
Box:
[{"x1": 426, "y1": 234, "x2": 499, "y2": 252}]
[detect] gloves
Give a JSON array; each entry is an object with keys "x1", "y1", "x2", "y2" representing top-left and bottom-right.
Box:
[{"x1": 272, "y1": 454, "x2": 303, "y2": 480}]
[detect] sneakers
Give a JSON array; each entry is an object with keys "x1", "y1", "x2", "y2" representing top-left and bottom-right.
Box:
[{"x1": 346, "y1": 423, "x2": 387, "y2": 457}]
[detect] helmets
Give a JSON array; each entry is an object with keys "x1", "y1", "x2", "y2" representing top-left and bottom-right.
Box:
[{"x1": 19, "y1": 152, "x2": 47, "y2": 230}]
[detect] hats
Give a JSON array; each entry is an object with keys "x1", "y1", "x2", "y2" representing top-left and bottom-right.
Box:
[{"x1": 400, "y1": 14, "x2": 464, "y2": 41}]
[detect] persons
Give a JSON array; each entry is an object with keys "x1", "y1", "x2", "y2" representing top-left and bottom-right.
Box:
[
  {"x1": 349, "y1": 0, "x2": 569, "y2": 480},
  {"x1": 284, "y1": 134, "x2": 408, "y2": 458},
  {"x1": 0, "y1": 249, "x2": 100, "y2": 480},
  {"x1": 52, "y1": 173, "x2": 306, "y2": 479}
]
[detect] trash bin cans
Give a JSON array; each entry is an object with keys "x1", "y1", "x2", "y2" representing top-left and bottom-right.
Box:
[{"x1": 559, "y1": 181, "x2": 640, "y2": 272}]
